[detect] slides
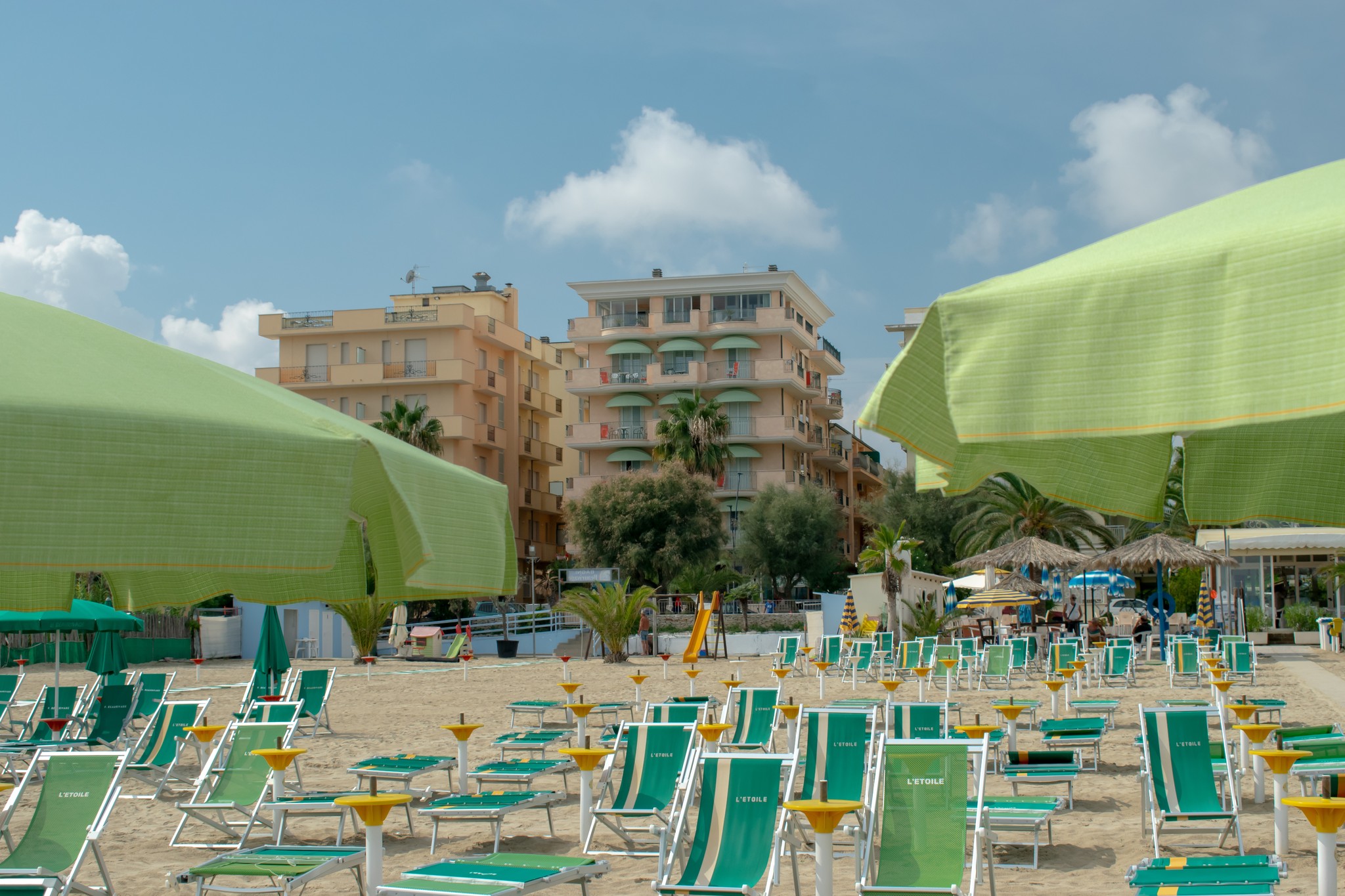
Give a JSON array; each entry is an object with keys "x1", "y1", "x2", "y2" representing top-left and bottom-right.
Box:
[
  {"x1": 442, "y1": 633, "x2": 467, "y2": 659},
  {"x1": 683, "y1": 590, "x2": 720, "y2": 664}
]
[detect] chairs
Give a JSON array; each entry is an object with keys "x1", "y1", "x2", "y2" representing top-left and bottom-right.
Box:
[
  {"x1": 1136, "y1": 702, "x2": 1247, "y2": 859},
  {"x1": 582, "y1": 719, "x2": 699, "y2": 856},
  {"x1": 650, "y1": 748, "x2": 802, "y2": 896},
  {"x1": 0, "y1": 746, "x2": 132, "y2": 896},
  {"x1": 769, "y1": 704, "x2": 879, "y2": 896},
  {"x1": 853, "y1": 733, "x2": 995, "y2": 896},
  {"x1": 1, "y1": 614, "x2": 1345, "y2": 800},
  {"x1": 166, "y1": 719, "x2": 301, "y2": 852}
]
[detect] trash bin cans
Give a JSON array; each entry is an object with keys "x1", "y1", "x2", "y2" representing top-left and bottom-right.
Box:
[
  {"x1": 1316, "y1": 617, "x2": 1337, "y2": 651},
  {"x1": 1340, "y1": 618, "x2": 1345, "y2": 649}
]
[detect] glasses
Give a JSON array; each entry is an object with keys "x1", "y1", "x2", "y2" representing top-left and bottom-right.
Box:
[
  {"x1": 1071, "y1": 597, "x2": 1077, "y2": 599},
  {"x1": 640, "y1": 613, "x2": 641, "y2": 614}
]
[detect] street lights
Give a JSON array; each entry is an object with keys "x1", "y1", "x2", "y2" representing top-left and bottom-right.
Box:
[{"x1": 525, "y1": 544, "x2": 539, "y2": 659}]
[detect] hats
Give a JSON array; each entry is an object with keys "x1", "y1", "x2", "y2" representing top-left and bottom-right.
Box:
[{"x1": 641, "y1": 610, "x2": 646, "y2": 615}]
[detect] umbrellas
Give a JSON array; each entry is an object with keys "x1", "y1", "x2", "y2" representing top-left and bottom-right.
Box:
[
  {"x1": 950, "y1": 537, "x2": 1094, "y2": 633},
  {"x1": 254, "y1": 605, "x2": 291, "y2": 696},
  {"x1": 0, "y1": 293, "x2": 522, "y2": 613},
  {"x1": 1196, "y1": 571, "x2": 1214, "y2": 640},
  {"x1": 1080, "y1": 533, "x2": 1239, "y2": 660},
  {"x1": 943, "y1": 567, "x2": 1137, "y2": 625},
  {"x1": 1, "y1": 597, "x2": 146, "y2": 738},
  {"x1": 840, "y1": 587, "x2": 862, "y2": 655},
  {"x1": 858, "y1": 158, "x2": 1345, "y2": 526}
]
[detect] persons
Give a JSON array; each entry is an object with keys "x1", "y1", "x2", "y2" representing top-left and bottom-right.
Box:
[
  {"x1": 1063, "y1": 594, "x2": 1082, "y2": 638},
  {"x1": 1132, "y1": 614, "x2": 1151, "y2": 643},
  {"x1": 1090, "y1": 618, "x2": 1114, "y2": 642}
]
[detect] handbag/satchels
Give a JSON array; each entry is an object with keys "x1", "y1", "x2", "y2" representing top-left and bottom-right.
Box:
[{"x1": 1065, "y1": 621, "x2": 1068, "y2": 628}]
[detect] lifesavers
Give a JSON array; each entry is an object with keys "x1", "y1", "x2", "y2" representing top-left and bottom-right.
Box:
[{"x1": 1147, "y1": 592, "x2": 1176, "y2": 620}]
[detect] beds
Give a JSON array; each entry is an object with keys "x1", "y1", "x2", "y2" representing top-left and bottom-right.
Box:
[
  {"x1": 260, "y1": 790, "x2": 432, "y2": 846},
  {"x1": 416, "y1": 789, "x2": 566, "y2": 854},
  {"x1": 175, "y1": 844, "x2": 383, "y2": 896},
  {"x1": 963, "y1": 795, "x2": 1067, "y2": 868},
  {"x1": 375, "y1": 853, "x2": 609, "y2": 896},
  {"x1": 1124, "y1": 854, "x2": 1289, "y2": 896}
]
